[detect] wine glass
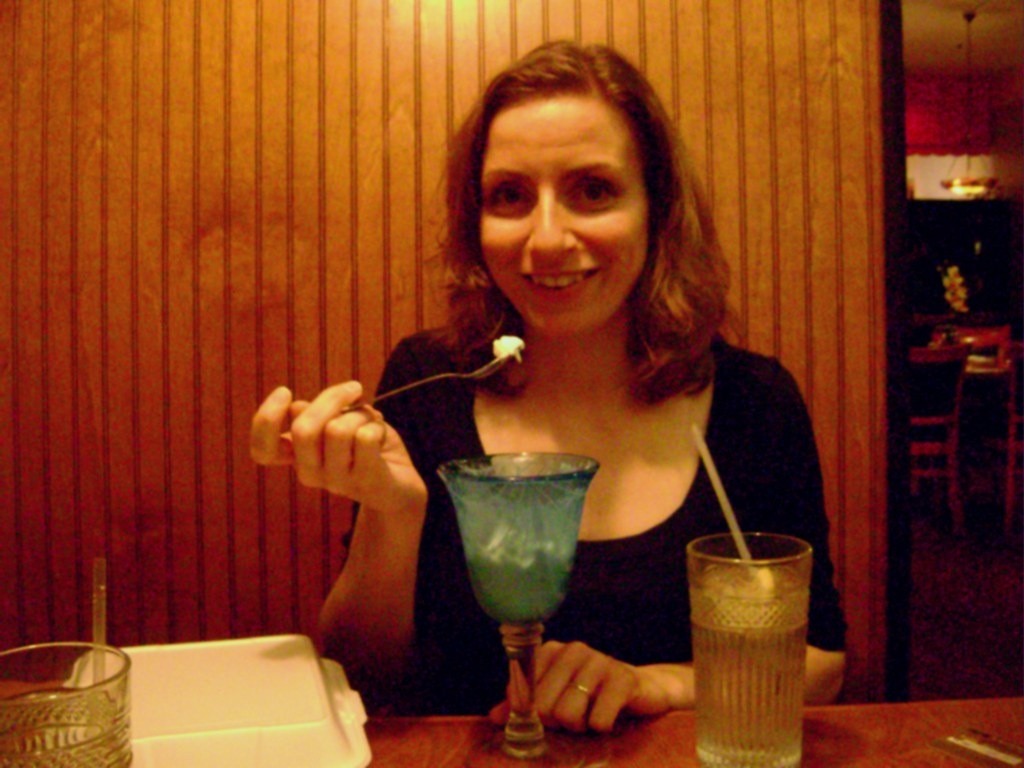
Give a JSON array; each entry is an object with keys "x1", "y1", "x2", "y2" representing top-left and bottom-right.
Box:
[{"x1": 436, "y1": 451, "x2": 603, "y2": 759}]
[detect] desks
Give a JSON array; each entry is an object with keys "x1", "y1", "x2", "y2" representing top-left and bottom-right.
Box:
[{"x1": 359, "y1": 694, "x2": 1024, "y2": 768}]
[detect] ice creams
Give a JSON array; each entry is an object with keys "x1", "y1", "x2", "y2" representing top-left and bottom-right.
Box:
[{"x1": 460, "y1": 496, "x2": 575, "y2": 621}]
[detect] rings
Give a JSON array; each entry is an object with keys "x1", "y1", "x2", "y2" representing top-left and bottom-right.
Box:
[{"x1": 571, "y1": 681, "x2": 591, "y2": 696}]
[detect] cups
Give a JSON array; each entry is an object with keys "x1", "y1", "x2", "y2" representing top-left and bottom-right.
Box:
[
  {"x1": 0, "y1": 641, "x2": 136, "y2": 768},
  {"x1": 685, "y1": 531, "x2": 813, "y2": 768}
]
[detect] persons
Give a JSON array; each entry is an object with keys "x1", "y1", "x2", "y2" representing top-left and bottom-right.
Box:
[{"x1": 249, "y1": 41, "x2": 848, "y2": 732}]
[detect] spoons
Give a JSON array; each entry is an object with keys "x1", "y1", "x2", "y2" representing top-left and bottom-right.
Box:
[{"x1": 342, "y1": 354, "x2": 509, "y2": 413}]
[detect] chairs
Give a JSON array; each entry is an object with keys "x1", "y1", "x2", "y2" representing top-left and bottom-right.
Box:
[{"x1": 907, "y1": 313, "x2": 1024, "y2": 538}]
